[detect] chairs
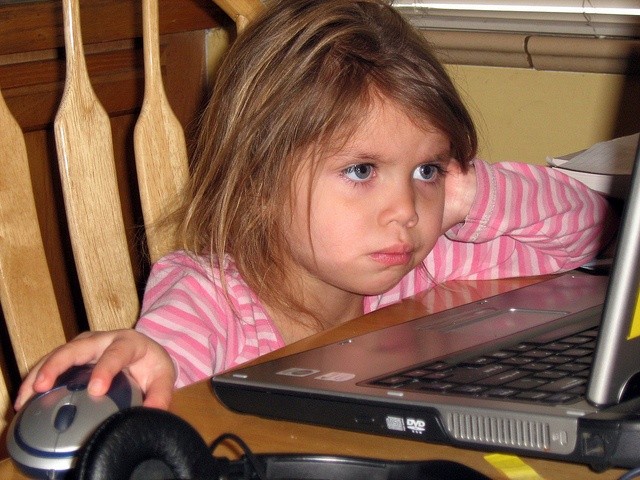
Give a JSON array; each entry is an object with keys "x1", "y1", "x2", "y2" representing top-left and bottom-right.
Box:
[{"x1": 0, "y1": 0, "x2": 273, "y2": 463}]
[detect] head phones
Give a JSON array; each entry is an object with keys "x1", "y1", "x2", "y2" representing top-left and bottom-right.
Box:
[{"x1": 74, "y1": 406, "x2": 492, "y2": 480}]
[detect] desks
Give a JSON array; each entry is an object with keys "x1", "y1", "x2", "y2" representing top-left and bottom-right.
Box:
[{"x1": 1, "y1": 268, "x2": 640, "y2": 479}]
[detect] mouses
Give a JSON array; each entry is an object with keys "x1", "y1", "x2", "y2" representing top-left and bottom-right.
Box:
[{"x1": 6, "y1": 365, "x2": 145, "y2": 480}]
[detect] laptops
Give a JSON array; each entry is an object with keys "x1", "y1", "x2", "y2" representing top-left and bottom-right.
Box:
[{"x1": 212, "y1": 137, "x2": 640, "y2": 473}]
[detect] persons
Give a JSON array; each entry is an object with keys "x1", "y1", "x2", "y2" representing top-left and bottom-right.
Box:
[{"x1": 14, "y1": 0, "x2": 619, "y2": 413}]
